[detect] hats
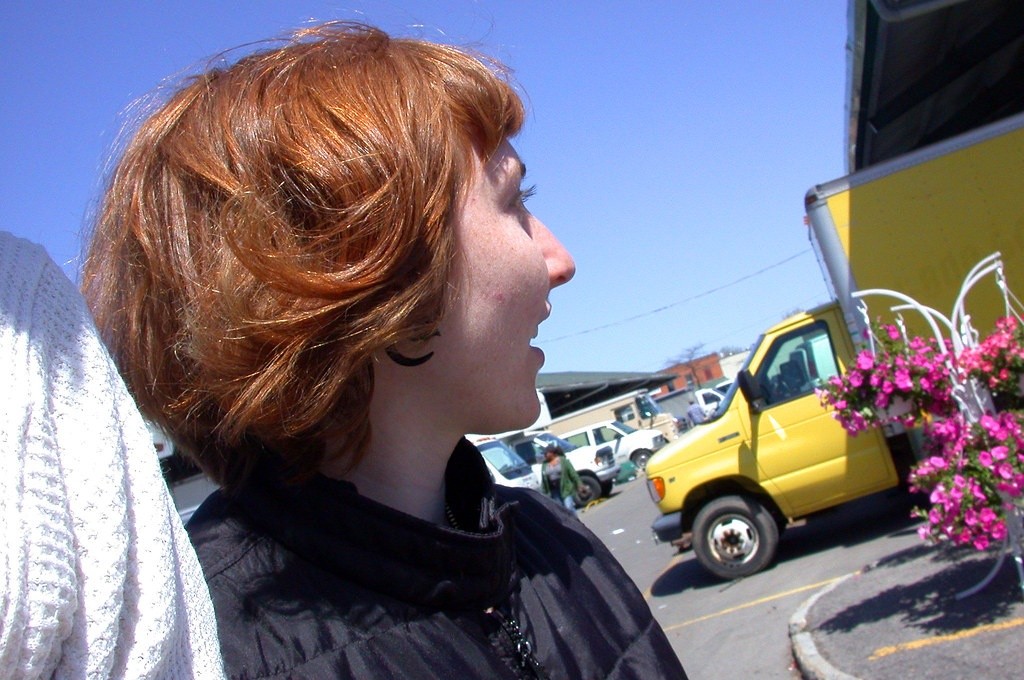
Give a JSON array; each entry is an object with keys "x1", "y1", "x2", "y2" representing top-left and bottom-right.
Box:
[{"x1": 541, "y1": 446, "x2": 556, "y2": 454}]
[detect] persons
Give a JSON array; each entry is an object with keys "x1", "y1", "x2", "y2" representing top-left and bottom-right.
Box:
[
  {"x1": 78, "y1": 21, "x2": 690, "y2": 680},
  {"x1": 541, "y1": 445, "x2": 586, "y2": 513},
  {"x1": 0, "y1": 229, "x2": 227, "y2": 680}
]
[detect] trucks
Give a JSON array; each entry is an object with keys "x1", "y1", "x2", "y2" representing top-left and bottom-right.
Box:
[
  {"x1": 465, "y1": 376, "x2": 736, "y2": 512},
  {"x1": 644, "y1": 104, "x2": 1024, "y2": 581}
]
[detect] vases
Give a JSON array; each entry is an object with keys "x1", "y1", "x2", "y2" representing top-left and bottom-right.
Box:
[{"x1": 869, "y1": 394, "x2": 914, "y2": 420}]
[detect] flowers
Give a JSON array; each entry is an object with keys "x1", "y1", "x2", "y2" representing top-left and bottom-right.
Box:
[{"x1": 815, "y1": 315, "x2": 1024, "y2": 551}]
